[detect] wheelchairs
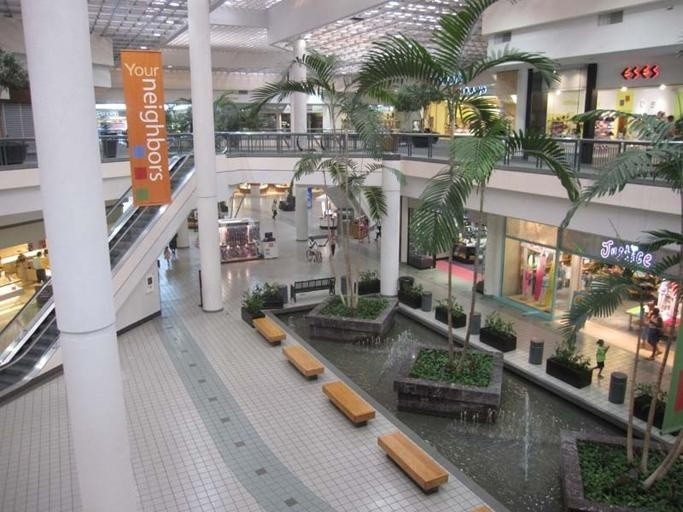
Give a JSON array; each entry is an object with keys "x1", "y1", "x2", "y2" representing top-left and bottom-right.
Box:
[{"x1": 305, "y1": 245, "x2": 322, "y2": 264}]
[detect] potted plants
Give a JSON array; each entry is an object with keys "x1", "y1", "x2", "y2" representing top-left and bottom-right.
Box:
[
  {"x1": 259, "y1": 281, "x2": 284, "y2": 310},
  {"x1": 240, "y1": 287, "x2": 266, "y2": 329},
  {"x1": 0, "y1": 42, "x2": 31, "y2": 168}
]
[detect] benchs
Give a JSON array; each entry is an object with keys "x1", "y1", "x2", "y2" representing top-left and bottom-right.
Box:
[
  {"x1": 320, "y1": 380, "x2": 376, "y2": 428},
  {"x1": 634, "y1": 393, "x2": 667, "y2": 433},
  {"x1": 398, "y1": 289, "x2": 422, "y2": 308},
  {"x1": 478, "y1": 326, "x2": 517, "y2": 352},
  {"x1": 436, "y1": 304, "x2": 467, "y2": 330},
  {"x1": 250, "y1": 316, "x2": 286, "y2": 347},
  {"x1": 406, "y1": 250, "x2": 434, "y2": 272},
  {"x1": 373, "y1": 429, "x2": 450, "y2": 495},
  {"x1": 354, "y1": 278, "x2": 381, "y2": 296},
  {"x1": 546, "y1": 355, "x2": 593, "y2": 390},
  {"x1": 288, "y1": 275, "x2": 336, "y2": 304},
  {"x1": 466, "y1": 501, "x2": 495, "y2": 512},
  {"x1": 281, "y1": 343, "x2": 325, "y2": 381}
]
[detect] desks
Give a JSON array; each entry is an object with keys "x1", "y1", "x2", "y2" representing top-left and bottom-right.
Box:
[{"x1": 624, "y1": 302, "x2": 646, "y2": 333}]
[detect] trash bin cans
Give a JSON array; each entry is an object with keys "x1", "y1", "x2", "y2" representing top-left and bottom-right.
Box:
[
  {"x1": 472, "y1": 312, "x2": 481, "y2": 335},
  {"x1": 529, "y1": 338, "x2": 544, "y2": 364},
  {"x1": 337, "y1": 211, "x2": 352, "y2": 235},
  {"x1": 421, "y1": 292, "x2": 432, "y2": 311},
  {"x1": 277, "y1": 285, "x2": 288, "y2": 305},
  {"x1": 609, "y1": 372, "x2": 628, "y2": 404},
  {"x1": 397, "y1": 276, "x2": 414, "y2": 289},
  {"x1": 101, "y1": 132, "x2": 118, "y2": 158}
]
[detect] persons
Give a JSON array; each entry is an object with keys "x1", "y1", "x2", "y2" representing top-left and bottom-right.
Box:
[
  {"x1": 307, "y1": 235, "x2": 324, "y2": 263},
  {"x1": 595, "y1": 113, "x2": 615, "y2": 135},
  {"x1": 271, "y1": 199, "x2": 278, "y2": 219},
  {"x1": 358, "y1": 219, "x2": 381, "y2": 244},
  {"x1": 587, "y1": 340, "x2": 610, "y2": 379},
  {"x1": 16, "y1": 248, "x2": 49, "y2": 282},
  {"x1": 657, "y1": 281, "x2": 668, "y2": 311},
  {"x1": 656, "y1": 110, "x2": 679, "y2": 140},
  {"x1": 162, "y1": 239, "x2": 177, "y2": 268},
  {"x1": 324, "y1": 230, "x2": 340, "y2": 260},
  {"x1": 641, "y1": 298, "x2": 664, "y2": 361}
]
